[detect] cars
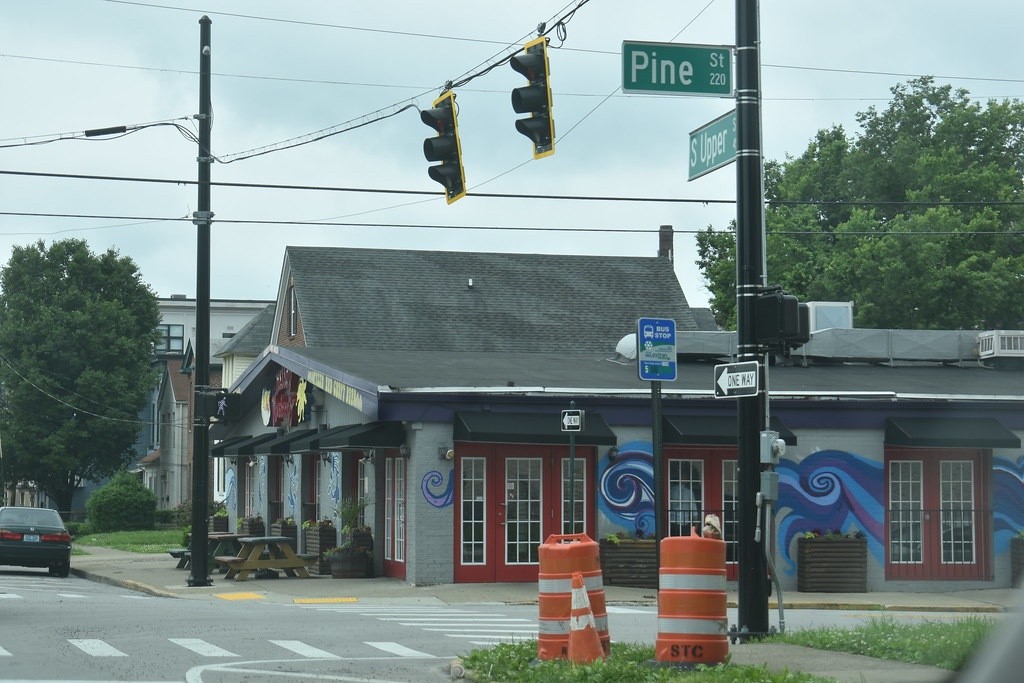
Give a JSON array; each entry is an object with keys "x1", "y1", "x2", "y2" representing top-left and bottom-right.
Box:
[{"x1": 0, "y1": 505, "x2": 72, "y2": 579}]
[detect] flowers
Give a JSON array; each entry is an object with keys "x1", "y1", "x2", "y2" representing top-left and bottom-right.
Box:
[
  {"x1": 804, "y1": 529, "x2": 867, "y2": 539},
  {"x1": 323, "y1": 543, "x2": 375, "y2": 560},
  {"x1": 301, "y1": 518, "x2": 334, "y2": 529},
  {"x1": 1014, "y1": 530, "x2": 1024, "y2": 543},
  {"x1": 275, "y1": 516, "x2": 294, "y2": 525},
  {"x1": 606, "y1": 529, "x2": 663, "y2": 544}
]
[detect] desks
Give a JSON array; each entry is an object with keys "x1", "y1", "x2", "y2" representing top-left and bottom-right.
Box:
[{"x1": 187, "y1": 531, "x2": 319, "y2": 581}]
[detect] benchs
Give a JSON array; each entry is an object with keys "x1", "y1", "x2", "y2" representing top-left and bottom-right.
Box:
[{"x1": 169, "y1": 548, "x2": 311, "y2": 581}]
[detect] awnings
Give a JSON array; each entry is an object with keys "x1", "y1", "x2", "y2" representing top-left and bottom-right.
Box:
[
  {"x1": 208, "y1": 421, "x2": 407, "y2": 458},
  {"x1": 884, "y1": 415, "x2": 1021, "y2": 449},
  {"x1": 661, "y1": 414, "x2": 797, "y2": 447},
  {"x1": 453, "y1": 409, "x2": 618, "y2": 446}
]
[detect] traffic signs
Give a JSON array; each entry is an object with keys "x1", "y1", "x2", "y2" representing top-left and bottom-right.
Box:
[
  {"x1": 560, "y1": 409, "x2": 582, "y2": 432},
  {"x1": 713, "y1": 360, "x2": 760, "y2": 400}
]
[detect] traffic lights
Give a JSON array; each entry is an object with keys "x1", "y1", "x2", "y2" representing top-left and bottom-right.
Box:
[
  {"x1": 509, "y1": 35, "x2": 556, "y2": 161},
  {"x1": 420, "y1": 90, "x2": 466, "y2": 205}
]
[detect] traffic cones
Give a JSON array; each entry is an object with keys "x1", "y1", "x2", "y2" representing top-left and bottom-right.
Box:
[{"x1": 567, "y1": 570, "x2": 607, "y2": 668}]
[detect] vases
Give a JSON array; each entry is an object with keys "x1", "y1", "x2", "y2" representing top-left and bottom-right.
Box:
[
  {"x1": 1010, "y1": 544, "x2": 1024, "y2": 591},
  {"x1": 797, "y1": 537, "x2": 867, "y2": 593},
  {"x1": 270, "y1": 523, "x2": 297, "y2": 558},
  {"x1": 304, "y1": 528, "x2": 336, "y2": 575},
  {"x1": 600, "y1": 538, "x2": 659, "y2": 589},
  {"x1": 330, "y1": 557, "x2": 367, "y2": 579}
]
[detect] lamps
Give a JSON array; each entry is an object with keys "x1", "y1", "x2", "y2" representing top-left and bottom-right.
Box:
[
  {"x1": 282, "y1": 455, "x2": 294, "y2": 465},
  {"x1": 230, "y1": 457, "x2": 236, "y2": 465},
  {"x1": 361, "y1": 450, "x2": 374, "y2": 460},
  {"x1": 321, "y1": 451, "x2": 332, "y2": 464},
  {"x1": 439, "y1": 447, "x2": 454, "y2": 460},
  {"x1": 400, "y1": 444, "x2": 411, "y2": 458}
]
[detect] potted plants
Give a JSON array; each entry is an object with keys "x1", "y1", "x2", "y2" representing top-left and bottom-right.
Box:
[
  {"x1": 330, "y1": 497, "x2": 374, "y2": 549},
  {"x1": 237, "y1": 516, "x2": 266, "y2": 538},
  {"x1": 208, "y1": 508, "x2": 228, "y2": 532}
]
